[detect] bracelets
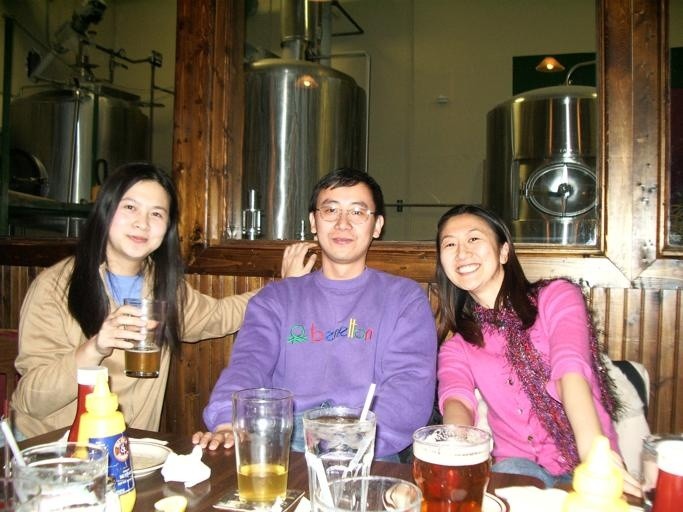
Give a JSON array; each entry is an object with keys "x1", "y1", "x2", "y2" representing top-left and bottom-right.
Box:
[{"x1": 94, "y1": 334, "x2": 113, "y2": 356}]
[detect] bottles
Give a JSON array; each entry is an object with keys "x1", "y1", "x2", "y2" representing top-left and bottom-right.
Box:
[
  {"x1": 650, "y1": 440, "x2": 682, "y2": 512},
  {"x1": 565, "y1": 437, "x2": 630, "y2": 511},
  {"x1": 66, "y1": 368, "x2": 108, "y2": 458},
  {"x1": 77, "y1": 374, "x2": 136, "y2": 512}
]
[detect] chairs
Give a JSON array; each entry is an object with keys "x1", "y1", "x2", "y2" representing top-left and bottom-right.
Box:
[
  {"x1": 0, "y1": 329, "x2": 167, "y2": 433},
  {"x1": 608, "y1": 360, "x2": 653, "y2": 427}
]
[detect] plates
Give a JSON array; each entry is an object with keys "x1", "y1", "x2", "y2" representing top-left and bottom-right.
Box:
[
  {"x1": 11, "y1": 439, "x2": 173, "y2": 479},
  {"x1": 381, "y1": 482, "x2": 510, "y2": 511}
]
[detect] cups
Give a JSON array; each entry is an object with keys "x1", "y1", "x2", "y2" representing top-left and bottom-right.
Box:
[
  {"x1": 230, "y1": 386, "x2": 294, "y2": 506},
  {"x1": 639, "y1": 434, "x2": 683, "y2": 507},
  {"x1": 302, "y1": 406, "x2": 376, "y2": 512},
  {"x1": 9, "y1": 441, "x2": 109, "y2": 511},
  {"x1": 313, "y1": 475, "x2": 423, "y2": 512},
  {"x1": 410, "y1": 423, "x2": 493, "y2": 512},
  {"x1": 123, "y1": 297, "x2": 166, "y2": 379}
]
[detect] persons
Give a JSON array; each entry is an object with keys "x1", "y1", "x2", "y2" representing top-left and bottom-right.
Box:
[
  {"x1": 193, "y1": 169, "x2": 438, "y2": 464},
  {"x1": 13, "y1": 162, "x2": 318, "y2": 441},
  {"x1": 431, "y1": 204, "x2": 645, "y2": 504}
]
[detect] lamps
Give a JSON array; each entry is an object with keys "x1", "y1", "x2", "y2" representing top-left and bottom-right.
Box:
[
  {"x1": 295, "y1": 74, "x2": 319, "y2": 90},
  {"x1": 536, "y1": 57, "x2": 565, "y2": 72}
]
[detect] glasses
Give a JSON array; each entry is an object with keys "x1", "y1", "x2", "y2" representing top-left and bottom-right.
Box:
[{"x1": 315, "y1": 206, "x2": 375, "y2": 225}]
